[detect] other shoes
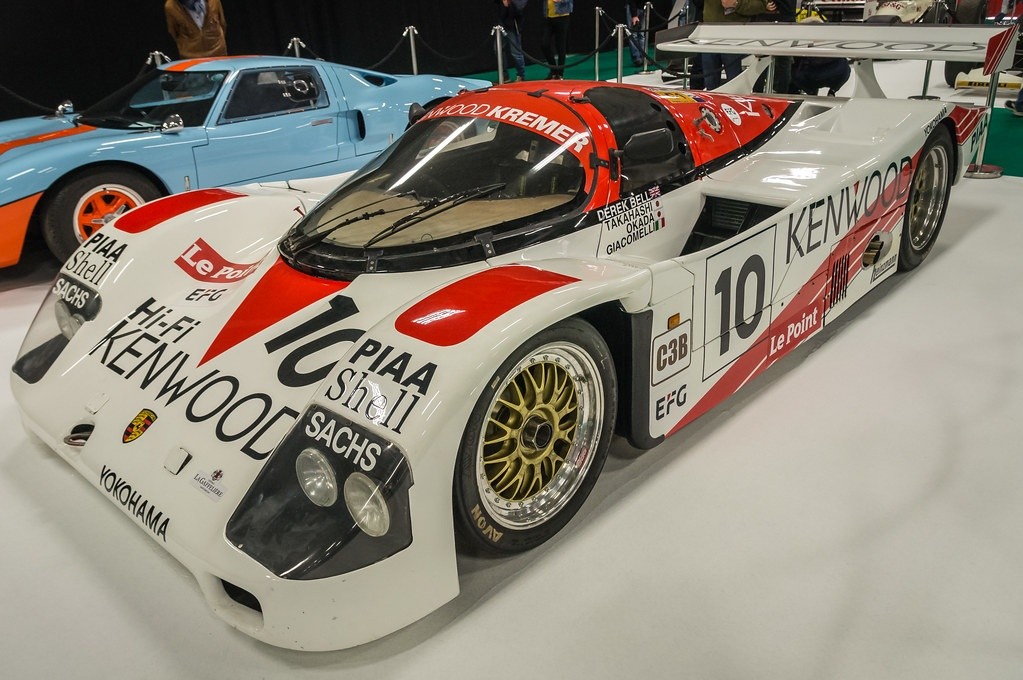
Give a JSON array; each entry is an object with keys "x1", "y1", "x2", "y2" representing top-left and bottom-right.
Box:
[
  {"x1": 1006, "y1": 101, "x2": 1023, "y2": 116},
  {"x1": 515, "y1": 75, "x2": 523, "y2": 81},
  {"x1": 635, "y1": 60, "x2": 643, "y2": 67},
  {"x1": 545, "y1": 73, "x2": 564, "y2": 80}
]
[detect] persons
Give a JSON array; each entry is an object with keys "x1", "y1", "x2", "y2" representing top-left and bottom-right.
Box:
[
  {"x1": 165, "y1": 0, "x2": 227, "y2": 89},
  {"x1": 689, "y1": 0, "x2": 850, "y2": 97},
  {"x1": 494, "y1": 0, "x2": 528, "y2": 84},
  {"x1": 624, "y1": 0, "x2": 653, "y2": 66},
  {"x1": 541, "y1": 0, "x2": 573, "y2": 80},
  {"x1": 1004, "y1": 15, "x2": 1023, "y2": 117}
]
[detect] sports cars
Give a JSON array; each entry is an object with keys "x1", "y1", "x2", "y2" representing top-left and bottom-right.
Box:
[
  {"x1": 0, "y1": 52, "x2": 479, "y2": 275},
  {"x1": 10, "y1": 18, "x2": 1022, "y2": 651}
]
